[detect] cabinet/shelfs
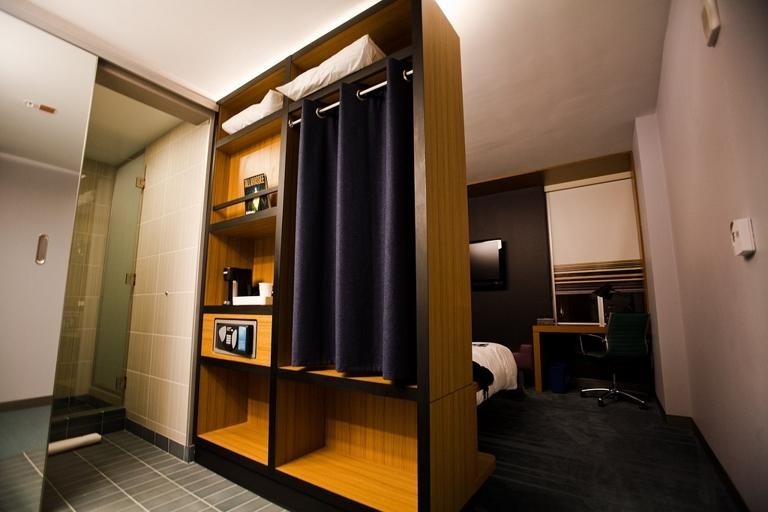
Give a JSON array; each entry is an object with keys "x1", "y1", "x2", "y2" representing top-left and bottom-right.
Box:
[{"x1": 192, "y1": 3, "x2": 498, "y2": 511}]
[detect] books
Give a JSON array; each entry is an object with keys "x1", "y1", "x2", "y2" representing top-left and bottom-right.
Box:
[{"x1": 244, "y1": 173, "x2": 272, "y2": 216}]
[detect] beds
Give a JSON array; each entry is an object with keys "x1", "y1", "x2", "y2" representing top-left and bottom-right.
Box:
[{"x1": 472, "y1": 342, "x2": 520, "y2": 416}]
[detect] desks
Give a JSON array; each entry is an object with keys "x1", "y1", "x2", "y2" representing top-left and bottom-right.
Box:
[{"x1": 531, "y1": 321, "x2": 607, "y2": 395}]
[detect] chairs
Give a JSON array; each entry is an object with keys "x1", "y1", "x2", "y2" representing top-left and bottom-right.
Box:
[{"x1": 579, "y1": 310, "x2": 653, "y2": 410}]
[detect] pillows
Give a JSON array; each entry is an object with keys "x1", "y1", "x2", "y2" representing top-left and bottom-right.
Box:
[{"x1": 220, "y1": 30, "x2": 388, "y2": 135}]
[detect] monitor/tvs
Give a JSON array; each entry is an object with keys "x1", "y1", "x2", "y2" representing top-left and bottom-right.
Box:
[{"x1": 468, "y1": 236, "x2": 507, "y2": 293}]
